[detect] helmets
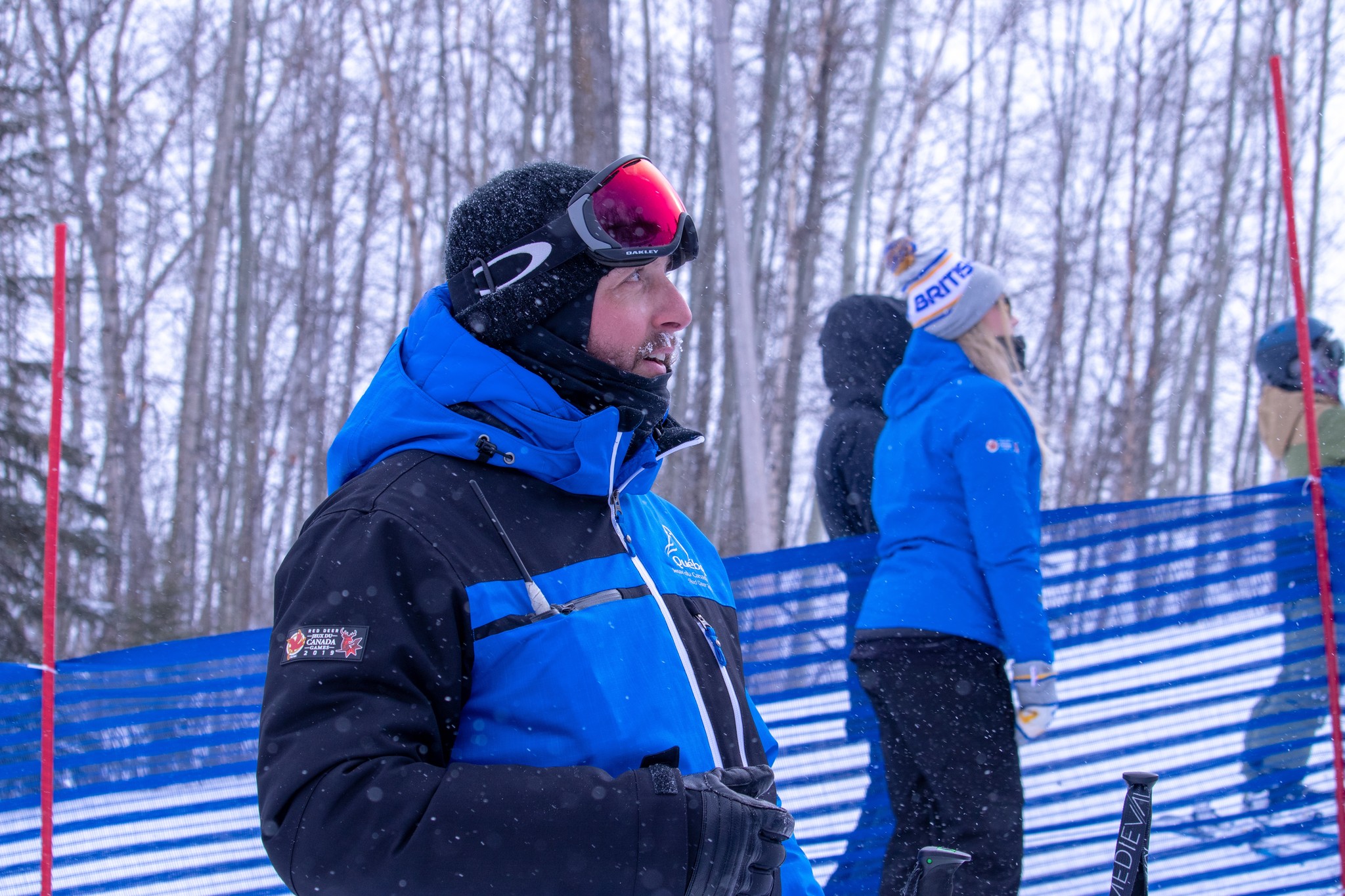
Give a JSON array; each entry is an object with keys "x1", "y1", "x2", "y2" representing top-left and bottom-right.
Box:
[{"x1": 1253, "y1": 315, "x2": 1333, "y2": 396}]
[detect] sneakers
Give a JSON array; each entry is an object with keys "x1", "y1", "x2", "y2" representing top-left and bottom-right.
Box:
[
  {"x1": 1242, "y1": 789, "x2": 1272, "y2": 812},
  {"x1": 1260, "y1": 783, "x2": 1338, "y2": 827}
]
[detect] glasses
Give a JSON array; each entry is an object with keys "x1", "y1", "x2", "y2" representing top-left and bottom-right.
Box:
[
  {"x1": 1322, "y1": 339, "x2": 1345, "y2": 372},
  {"x1": 447, "y1": 152, "x2": 700, "y2": 316}
]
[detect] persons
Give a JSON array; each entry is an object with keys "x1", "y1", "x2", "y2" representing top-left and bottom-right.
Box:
[
  {"x1": 1240, "y1": 316, "x2": 1345, "y2": 828},
  {"x1": 850, "y1": 237, "x2": 1058, "y2": 896},
  {"x1": 814, "y1": 293, "x2": 913, "y2": 896},
  {"x1": 255, "y1": 154, "x2": 822, "y2": 896}
]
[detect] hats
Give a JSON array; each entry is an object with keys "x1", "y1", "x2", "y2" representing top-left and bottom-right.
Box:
[
  {"x1": 443, "y1": 160, "x2": 620, "y2": 349},
  {"x1": 885, "y1": 238, "x2": 1003, "y2": 341}
]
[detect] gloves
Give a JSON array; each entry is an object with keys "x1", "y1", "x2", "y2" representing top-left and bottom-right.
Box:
[
  {"x1": 680, "y1": 763, "x2": 796, "y2": 896},
  {"x1": 1011, "y1": 659, "x2": 1058, "y2": 747}
]
